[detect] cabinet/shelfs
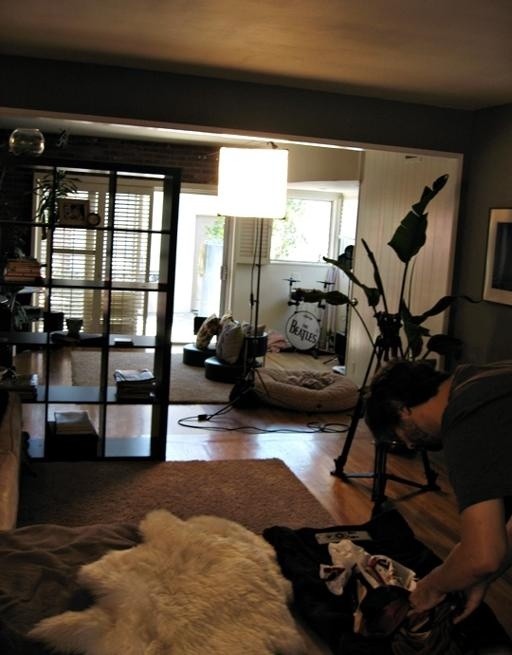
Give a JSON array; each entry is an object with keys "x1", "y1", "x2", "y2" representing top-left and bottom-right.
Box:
[{"x1": 1, "y1": 152, "x2": 183, "y2": 462}]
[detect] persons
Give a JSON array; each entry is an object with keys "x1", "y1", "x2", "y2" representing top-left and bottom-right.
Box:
[{"x1": 362, "y1": 358, "x2": 511, "y2": 624}]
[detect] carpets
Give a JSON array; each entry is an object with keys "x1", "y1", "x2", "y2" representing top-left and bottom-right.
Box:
[
  {"x1": 18, "y1": 458, "x2": 341, "y2": 537},
  {"x1": 71, "y1": 350, "x2": 333, "y2": 406}
]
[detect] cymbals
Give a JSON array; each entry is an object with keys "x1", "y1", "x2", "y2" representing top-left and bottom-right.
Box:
[
  {"x1": 317, "y1": 281, "x2": 335, "y2": 285},
  {"x1": 283, "y1": 280, "x2": 300, "y2": 282}
]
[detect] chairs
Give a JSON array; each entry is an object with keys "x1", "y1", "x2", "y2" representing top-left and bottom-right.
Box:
[{"x1": 183, "y1": 316, "x2": 268, "y2": 383}]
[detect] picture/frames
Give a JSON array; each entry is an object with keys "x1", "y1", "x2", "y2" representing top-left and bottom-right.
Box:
[{"x1": 481, "y1": 207, "x2": 511, "y2": 307}]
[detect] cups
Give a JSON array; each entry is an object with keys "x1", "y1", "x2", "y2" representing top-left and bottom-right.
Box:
[{"x1": 66, "y1": 317, "x2": 82, "y2": 332}]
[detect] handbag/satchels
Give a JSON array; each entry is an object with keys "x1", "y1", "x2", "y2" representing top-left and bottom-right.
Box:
[{"x1": 380, "y1": 597, "x2": 466, "y2": 654}]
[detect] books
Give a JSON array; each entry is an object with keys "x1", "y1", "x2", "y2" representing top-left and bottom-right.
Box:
[
  {"x1": 55, "y1": 412, "x2": 95, "y2": 435},
  {"x1": 3, "y1": 258, "x2": 42, "y2": 282},
  {"x1": 113, "y1": 368, "x2": 156, "y2": 400}
]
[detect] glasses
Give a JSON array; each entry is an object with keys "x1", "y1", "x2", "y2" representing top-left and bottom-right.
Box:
[{"x1": 382, "y1": 402, "x2": 407, "y2": 446}]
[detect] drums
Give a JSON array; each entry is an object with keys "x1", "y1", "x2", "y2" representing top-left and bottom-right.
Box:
[
  {"x1": 304, "y1": 288, "x2": 324, "y2": 303},
  {"x1": 285, "y1": 311, "x2": 321, "y2": 351},
  {"x1": 290, "y1": 288, "x2": 306, "y2": 302}
]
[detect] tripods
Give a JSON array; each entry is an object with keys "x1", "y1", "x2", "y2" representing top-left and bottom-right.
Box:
[{"x1": 334, "y1": 335, "x2": 440, "y2": 521}]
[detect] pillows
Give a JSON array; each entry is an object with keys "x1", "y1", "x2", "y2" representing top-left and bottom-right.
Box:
[{"x1": 194, "y1": 312, "x2": 266, "y2": 365}]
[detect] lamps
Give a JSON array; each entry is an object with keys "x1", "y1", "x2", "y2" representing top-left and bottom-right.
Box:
[{"x1": 206, "y1": 146, "x2": 289, "y2": 422}]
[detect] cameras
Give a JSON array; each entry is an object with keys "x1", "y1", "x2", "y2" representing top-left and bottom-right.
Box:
[{"x1": 375, "y1": 312, "x2": 402, "y2": 335}]
